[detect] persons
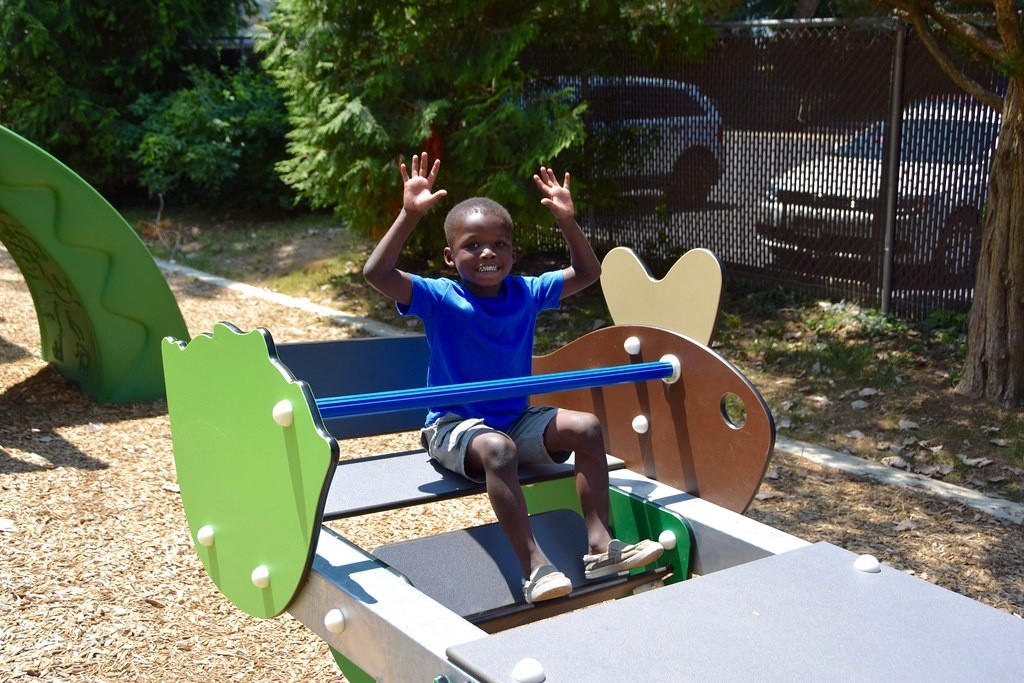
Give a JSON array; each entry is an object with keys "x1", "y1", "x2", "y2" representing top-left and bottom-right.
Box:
[{"x1": 363, "y1": 152, "x2": 665, "y2": 605}]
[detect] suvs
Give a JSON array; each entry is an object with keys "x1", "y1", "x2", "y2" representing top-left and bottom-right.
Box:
[{"x1": 506, "y1": 76, "x2": 731, "y2": 205}]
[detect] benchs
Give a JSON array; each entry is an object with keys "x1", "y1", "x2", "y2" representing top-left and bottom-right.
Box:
[{"x1": 276, "y1": 334, "x2": 626, "y2": 521}]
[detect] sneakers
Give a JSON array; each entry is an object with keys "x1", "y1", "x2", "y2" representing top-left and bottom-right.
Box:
[
  {"x1": 582, "y1": 538, "x2": 664, "y2": 580},
  {"x1": 521, "y1": 563, "x2": 573, "y2": 604}
]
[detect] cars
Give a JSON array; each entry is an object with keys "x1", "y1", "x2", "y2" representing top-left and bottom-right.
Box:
[{"x1": 755, "y1": 100, "x2": 1005, "y2": 278}]
